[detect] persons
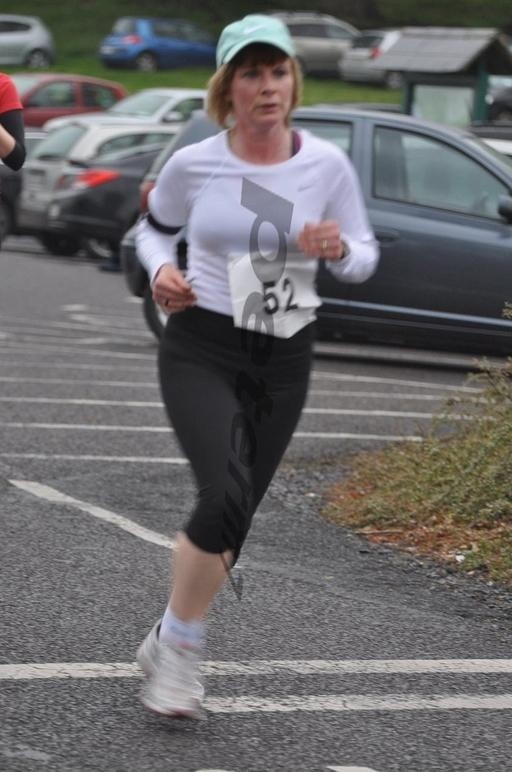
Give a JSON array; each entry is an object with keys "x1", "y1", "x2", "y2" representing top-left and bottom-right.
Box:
[
  {"x1": 0, "y1": 73, "x2": 26, "y2": 171},
  {"x1": 136, "y1": 15, "x2": 380, "y2": 718}
]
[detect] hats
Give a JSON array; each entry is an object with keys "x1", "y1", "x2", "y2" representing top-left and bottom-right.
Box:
[{"x1": 216, "y1": 14, "x2": 295, "y2": 68}]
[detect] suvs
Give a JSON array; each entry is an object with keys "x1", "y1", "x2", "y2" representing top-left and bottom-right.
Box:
[{"x1": 266, "y1": 13, "x2": 362, "y2": 81}]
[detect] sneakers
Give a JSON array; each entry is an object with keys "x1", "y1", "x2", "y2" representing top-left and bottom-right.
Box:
[{"x1": 136, "y1": 622, "x2": 205, "y2": 717}]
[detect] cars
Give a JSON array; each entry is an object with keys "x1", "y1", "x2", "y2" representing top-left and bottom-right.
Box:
[
  {"x1": 478, "y1": 74, "x2": 511, "y2": 116},
  {"x1": 0, "y1": 14, "x2": 56, "y2": 72},
  {"x1": 2, "y1": 73, "x2": 510, "y2": 272},
  {"x1": 98, "y1": 16, "x2": 217, "y2": 75},
  {"x1": 337, "y1": 27, "x2": 417, "y2": 90},
  {"x1": 116, "y1": 105, "x2": 511, "y2": 359}
]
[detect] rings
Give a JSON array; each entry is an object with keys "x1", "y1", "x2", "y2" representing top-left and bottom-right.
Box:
[
  {"x1": 319, "y1": 240, "x2": 328, "y2": 250},
  {"x1": 164, "y1": 299, "x2": 169, "y2": 306}
]
